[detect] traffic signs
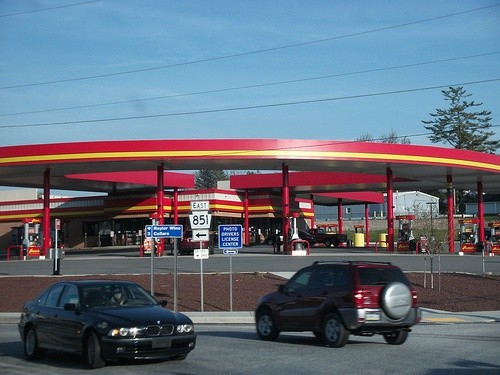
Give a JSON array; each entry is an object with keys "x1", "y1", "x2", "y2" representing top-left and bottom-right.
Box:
[{"x1": 192, "y1": 229, "x2": 209, "y2": 240}]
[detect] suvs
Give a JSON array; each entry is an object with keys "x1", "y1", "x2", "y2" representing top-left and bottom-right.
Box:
[{"x1": 253, "y1": 261, "x2": 425, "y2": 350}]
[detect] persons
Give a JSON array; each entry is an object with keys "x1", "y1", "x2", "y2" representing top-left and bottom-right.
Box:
[
  {"x1": 276, "y1": 234, "x2": 280, "y2": 252},
  {"x1": 107, "y1": 286, "x2": 130, "y2": 307},
  {"x1": 272, "y1": 235, "x2": 276, "y2": 254}
]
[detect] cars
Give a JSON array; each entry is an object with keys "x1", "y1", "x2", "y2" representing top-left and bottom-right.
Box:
[
  {"x1": 265, "y1": 229, "x2": 316, "y2": 246},
  {"x1": 476, "y1": 234, "x2": 500, "y2": 252},
  {"x1": 18, "y1": 280, "x2": 197, "y2": 369},
  {"x1": 179, "y1": 230, "x2": 216, "y2": 255}
]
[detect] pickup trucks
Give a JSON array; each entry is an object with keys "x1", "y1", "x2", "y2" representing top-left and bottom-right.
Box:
[{"x1": 310, "y1": 228, "x2": 346, "y2": 248}]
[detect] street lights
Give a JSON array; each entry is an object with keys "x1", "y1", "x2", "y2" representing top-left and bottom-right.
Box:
[{"x1": 427, "y1": 201, "x2": 437, "y2": 245}]
